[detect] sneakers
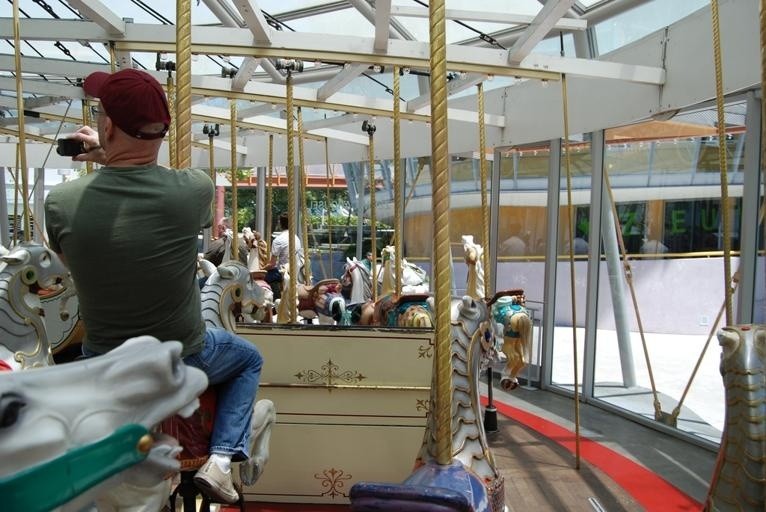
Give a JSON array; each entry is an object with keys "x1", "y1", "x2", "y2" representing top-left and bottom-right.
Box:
[{"x1": 195, "y1": 463, "x2": 239, "y2": 503}]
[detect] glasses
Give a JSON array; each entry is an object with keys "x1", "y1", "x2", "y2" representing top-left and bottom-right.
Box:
[{"x1": 91, "y1": 106, "x2": 104, "y2": 116}]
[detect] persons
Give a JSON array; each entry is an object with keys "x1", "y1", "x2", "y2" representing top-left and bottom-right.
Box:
[
  {"x1": 43, "y1": 68, "x2": 263, "y2": 501},
  {"x1": 199, "y1": 216, "x2": 248, "y2": 291},
  {"x1": 260, "y1": 213, "x2": 304, "y2": 315},
  {"x1": 361, "y1": 251, "x2": 372, "y2": 273},
  {"x1": 565, "y1": 228, "x2": 587, "y2": 255},
  {"x1": 370, "y1": 260, "x2": 385, "y2": 295},
  {"x1": 500, "y1": 223, "x2": 527, "y2": 258},
  {"x1": 641, "y1": 227, "x2": 668, "y2": 258}
]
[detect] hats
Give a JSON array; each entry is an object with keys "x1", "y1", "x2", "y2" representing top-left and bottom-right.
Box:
[{"x1": 85, "y1": 68, "x2": 171, "y2": 139}]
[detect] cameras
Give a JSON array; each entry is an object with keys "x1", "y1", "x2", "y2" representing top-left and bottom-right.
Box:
[{"x1": 56, "y1": 138, "x2": 90, "y2": 156}]
